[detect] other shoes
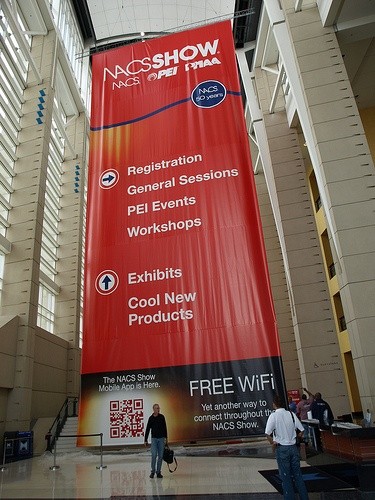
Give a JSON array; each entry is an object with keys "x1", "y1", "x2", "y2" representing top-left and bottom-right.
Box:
[
  {"x1": 150, "y1": 471, "x2": 155, "y2": 478},
  {"x1": 157, "y1": 472, "x2": 163, "y2": 478}
]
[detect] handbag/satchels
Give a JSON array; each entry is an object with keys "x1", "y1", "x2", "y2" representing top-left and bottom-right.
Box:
[
  {"x1": 162, "y1": 444, "x2": 178, "y2": 473},
  {"x1": 289, "y1": 410, "x2": 305, "y2": 448}
]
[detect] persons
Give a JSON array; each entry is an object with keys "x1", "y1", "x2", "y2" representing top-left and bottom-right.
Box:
[
  {"x1": 144, "y1": 404, "x2": 168, "y2": 478},
  {"x1": 265, "y1": 396, "x2": 309, "y2": 500},
  {"x1": 288, "y1": 397, "x2": 296, "y2": 414},
  {"x1": 297, "y1": 388, "x2": 315, "y2": 446},
  {"x1": 309, "y1": 392, "x2": 334, "y2": 454}
]
